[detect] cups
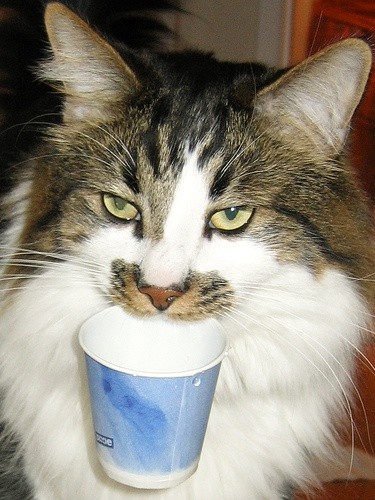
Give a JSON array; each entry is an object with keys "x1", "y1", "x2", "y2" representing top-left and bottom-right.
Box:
[{"x1": 78, "y1": 304, "x2": 229, "y2": 491}]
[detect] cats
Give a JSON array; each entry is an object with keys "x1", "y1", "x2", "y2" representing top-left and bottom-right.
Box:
[{"x1": 0, "y1": 2, "x2": 374, "y2": 499}]
